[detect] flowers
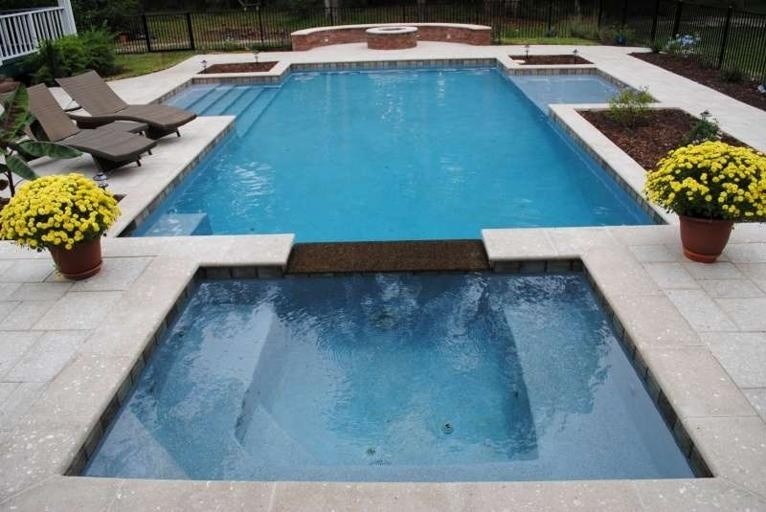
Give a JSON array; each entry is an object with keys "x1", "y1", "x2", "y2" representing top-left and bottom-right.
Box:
[
  {"x1": 0, "y1": 172, "x2": 122, "y2": 251},
  {"x1": 645, "y1": 139, "x2": 765, "y2": 219}
]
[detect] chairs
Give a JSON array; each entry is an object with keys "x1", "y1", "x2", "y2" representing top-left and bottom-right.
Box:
[{"x1": 25, "y1": 70, "x2": 197, "y2": 175}]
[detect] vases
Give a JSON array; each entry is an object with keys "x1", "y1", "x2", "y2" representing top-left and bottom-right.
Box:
[
  {"x1": 43, "y1": 237, "x2": 104, "y2": 278},
  {"x1": 678, "y1": 213, "x2": 735, "y2": 262}
]
